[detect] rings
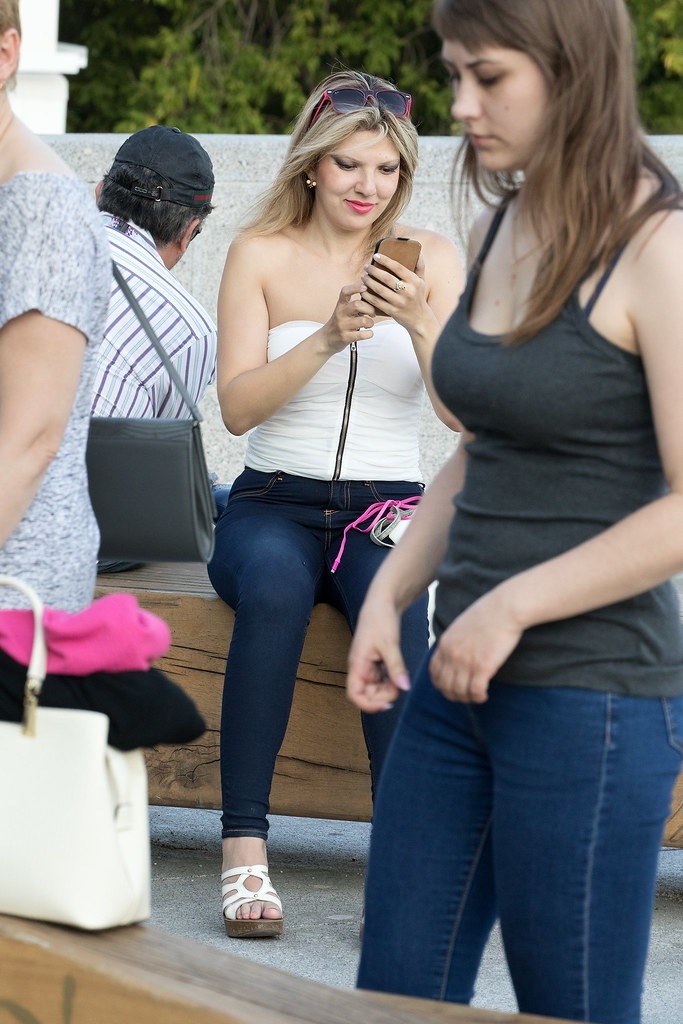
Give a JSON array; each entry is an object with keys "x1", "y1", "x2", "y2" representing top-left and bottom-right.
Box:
[{"x1": 393, "y1": 280, "x2": 405, "y2": 292}]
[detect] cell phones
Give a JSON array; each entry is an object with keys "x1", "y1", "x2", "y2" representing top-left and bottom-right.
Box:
[{"x1": 362, "y1": 235, "x2": 422, "y2": 317}]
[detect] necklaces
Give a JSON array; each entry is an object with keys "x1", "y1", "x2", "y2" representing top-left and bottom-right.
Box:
[{"x1": 511, "y1": 197, "x2": 551, "y2": 280}]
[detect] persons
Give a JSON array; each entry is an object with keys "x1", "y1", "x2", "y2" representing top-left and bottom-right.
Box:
[
  {"x1": 205, "y1": 71, "x2": 465, "y2": 937},
  {"x1": 0, "y1": 0, "x2": 112, "y2": 615},
  {"x1": 347, "y1": 0, "x2": 683, "y2": 1024},
  {"x1": 90, "y1": 125, "x2": 231, "y2": 573}
]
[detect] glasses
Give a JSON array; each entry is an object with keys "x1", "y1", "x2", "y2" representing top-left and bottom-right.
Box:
[{"x1": 309, "y1": 88, "x2": 412, "y2": 128}]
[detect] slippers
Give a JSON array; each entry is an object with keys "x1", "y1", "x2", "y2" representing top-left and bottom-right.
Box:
[{"x1": 219, "y1": 865, "x2": 284, "y2": 937}]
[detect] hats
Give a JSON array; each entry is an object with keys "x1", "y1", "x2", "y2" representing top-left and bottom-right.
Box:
[{"x1": 102, "y1": 124, "x2": 216, "y2": 207}]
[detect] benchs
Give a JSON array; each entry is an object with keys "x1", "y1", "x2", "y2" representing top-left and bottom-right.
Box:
[{"x1": 89, "y1": 564, "x2": 683, "y2": 850}]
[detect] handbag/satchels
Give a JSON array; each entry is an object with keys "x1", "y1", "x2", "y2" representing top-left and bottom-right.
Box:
[
  {"x1": 374, "y1": 507, "x2": 437, "y2": 652},
  {"x1": 82, "y1": 258, "x2": 220, "y2": 563},
  {"x1": 0, "y1": 575, "x2": 152, "y2": 931}
]
